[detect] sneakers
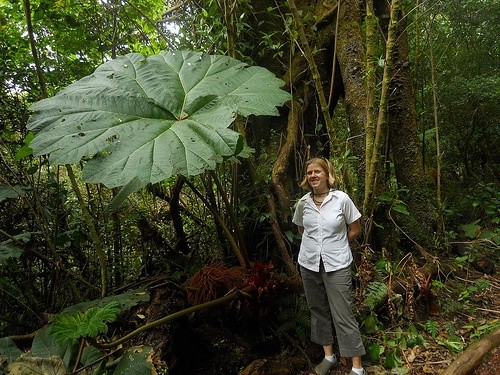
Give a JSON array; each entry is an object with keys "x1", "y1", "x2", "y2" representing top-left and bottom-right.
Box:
[
  {"x1": 348, "y1": 369, "x2": 366, "y2": 375},
  {"x1": 314, "y1": 353, "x2": 338, "y2": 375}
]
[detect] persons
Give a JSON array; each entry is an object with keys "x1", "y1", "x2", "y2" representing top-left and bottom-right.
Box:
[{"x1": 291, "y1": 156, "x2": 369, "y2": 375}]
